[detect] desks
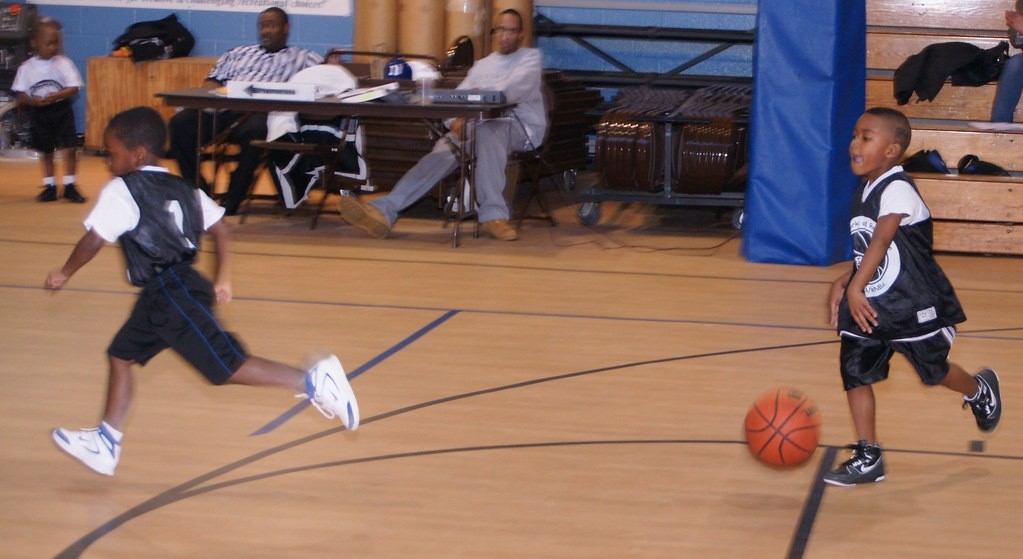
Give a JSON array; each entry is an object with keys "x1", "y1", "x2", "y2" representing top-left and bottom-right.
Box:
[{"x1": 153, "y1": 86, "x2": 519, "y2": 249}]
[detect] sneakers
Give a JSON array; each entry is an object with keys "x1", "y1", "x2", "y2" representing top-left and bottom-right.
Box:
[
  {"x1": 962, "y1": 367, "x2": 1001, "y2": 433},
  {"x1": 337, "y1": 195, "x2": 388, "y2": 239},
  {"x1": 824, "y1": 439, "x2": 884, "y2": 485},
  {"x1": 295, "y1": 356, "x2": 358, "y2": 431},
  {"x1": 478, "y1": 219, "x2": 518, "y2": 241},
  {"x1": 51, "y1": 418, "x2": 126, "y2": 476}
]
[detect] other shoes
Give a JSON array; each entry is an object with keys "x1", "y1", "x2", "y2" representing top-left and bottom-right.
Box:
[
  {"x1": 61, "y1": 184, "x2": 84, "y2": 201},
  {"x1": 36, "y1": 183, "x2": 57, "y2": 201}
]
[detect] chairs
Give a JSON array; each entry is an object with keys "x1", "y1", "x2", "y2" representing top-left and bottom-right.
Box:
[{"x1": 200, "y1": 59, "x2": 570, "y2": 232}]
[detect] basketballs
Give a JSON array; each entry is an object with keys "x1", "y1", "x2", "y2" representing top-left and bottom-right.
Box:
[{"x1": 745, "y1": 388, "x2": 820, "y2": 468}]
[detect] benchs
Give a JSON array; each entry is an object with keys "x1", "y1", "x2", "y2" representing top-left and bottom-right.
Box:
[{"x1": 864, "y1": 23, "x2": 1023, "y2": 256}]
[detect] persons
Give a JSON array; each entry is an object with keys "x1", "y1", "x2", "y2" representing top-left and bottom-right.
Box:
[
  {"x1": 823, "y1": 108, "x2": 1002, "y2": 487},
  {"x1": 990, "y1": 0, "x2": 1023, "y2": 123},
  {"x1": 9, "y1": 20, "x2": 87, "y2": 203},
  {"x1": 338, "y1": 10, "x2": 548, "y2": 242},
  {"x1": 167, "y1": 6, "x2": 324, "y2": 215},
  {"x1": 44, "y1": 107, "x2": 359, "y2": 475}
]
